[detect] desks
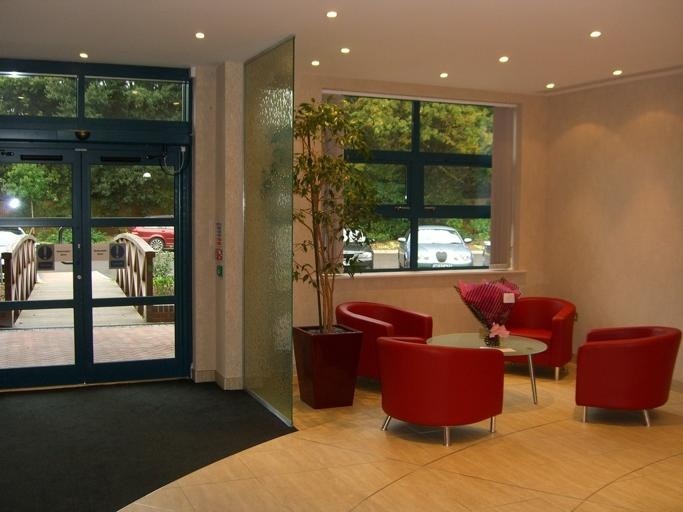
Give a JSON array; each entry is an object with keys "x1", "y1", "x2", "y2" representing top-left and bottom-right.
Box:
[{"x1": 424, "y1": 332, "x2": 550, "y2": 410}]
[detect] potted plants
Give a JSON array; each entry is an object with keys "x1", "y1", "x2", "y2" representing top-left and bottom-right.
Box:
[{"x1": 256, "y1": 91, "x2": 382, "y2": 410}]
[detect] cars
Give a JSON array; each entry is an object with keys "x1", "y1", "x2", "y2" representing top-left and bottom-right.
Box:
[
  {"x1": 480, "y1": 238, "x2": 490, "y2": 266},
  {"x1": 127, "y1": 215, "x2": 174, "y2": 252},
  {"x1": 395, "y1": 225, "x2": 472, "y2": 270},
  {"x1": 0, "y1": 226, "x2": 39, "y2": 257},
  {"x1": 341, "y1": 226, "x2": 374, "y2": 272}
]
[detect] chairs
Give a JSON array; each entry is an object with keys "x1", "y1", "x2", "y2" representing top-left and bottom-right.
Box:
[
  {"x1": 373, "y1": 333, "x2": 506, "y2": 448},
  {"x1": 573, "y1": 322, "x2": 682, "y2": 429},
  {"x1": 494, "y1": 294, "x2": 578, "y2": 384},
  {"x1": 334, "y1": 298, "x2": 434, "y2": 394}
]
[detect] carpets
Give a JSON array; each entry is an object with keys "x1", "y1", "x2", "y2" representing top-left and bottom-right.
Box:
[{"x1": 0, "y1": 370, "x2": 301, "y2": 512}]
